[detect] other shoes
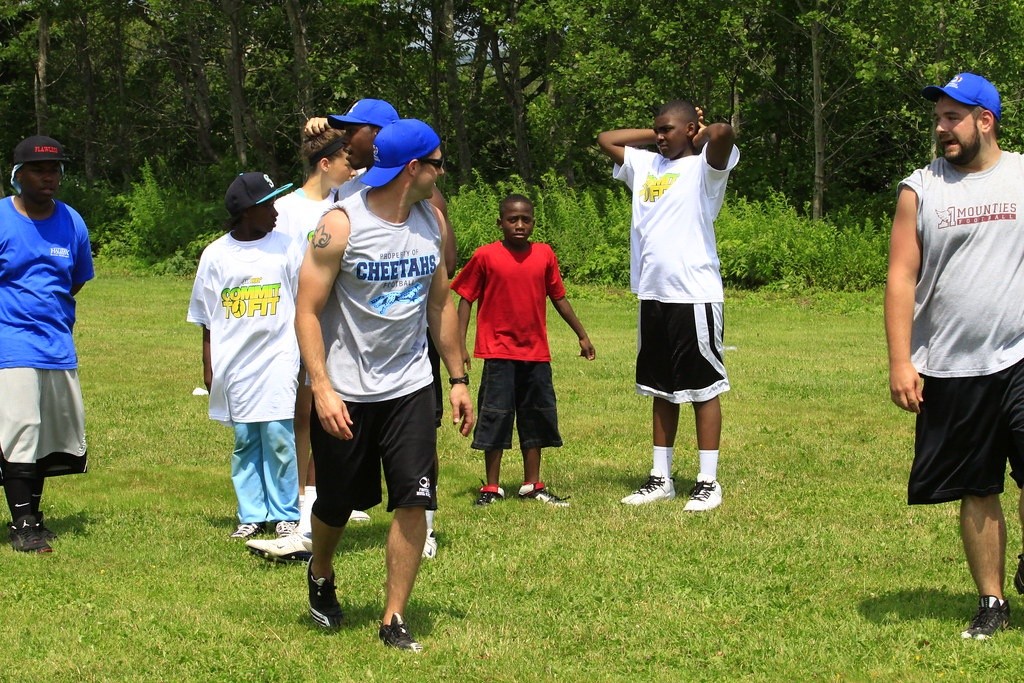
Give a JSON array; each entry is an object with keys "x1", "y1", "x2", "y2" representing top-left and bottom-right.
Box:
[
  {"x1": 229, "y1": 522, "x2": 269, "y2": 542},
  {"x1": 276, "y1": 522, "x2": 298, "y2": 537}
]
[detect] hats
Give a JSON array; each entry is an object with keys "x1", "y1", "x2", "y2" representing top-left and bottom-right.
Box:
[
  {"x1": 223, "y1": 170, "x2": 294, "y2": 215},
  {"x1": 11, "y1": 136, "x2": 75, "y2": 166},
  {"x1": 326, "y1": 97, "x2": 400, "y2": 130},
  {"x1": 924, "y1": 72, "x2": 1001, "y2": 122},
  {"x1": 357, "y1": 118, "x2": 440, "y2": 189}
]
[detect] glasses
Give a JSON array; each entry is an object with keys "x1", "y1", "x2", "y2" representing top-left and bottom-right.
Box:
[{"x1": 418, "y1": 157, "x2": 445, "y2": 168}]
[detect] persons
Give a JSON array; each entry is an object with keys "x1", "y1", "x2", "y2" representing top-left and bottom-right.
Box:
[
  {"x1": 451, "y1": 194, "x2": 595, "y2": 509},
  {"x1": 597, "y1": 100, "x2": 741, "y2": 512},
  {"x1": 295, "y1": 118, "x2": 475, "y2": 652},
  {"x1": 244, "y1": 100, "x2": 456, "y2": 558},
  {"x1": 186, "y1": 172, "x2": 307, "y2": 538},
  {"x1": 0, "y1": 136, "x2": 96, "y2": 551},
  {"x1": 883, "y1": 75, "x2": 1024, "y2": 640}
]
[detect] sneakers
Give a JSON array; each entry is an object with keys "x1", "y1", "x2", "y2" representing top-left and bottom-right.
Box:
[
  {"x1": 377, "y1": 612, "x2": 424, "y2": 651},
  {"x1": 305, "y1": 555, "x2": 345, "y2": 632},
  {"x1": 244, "y1": 532, "x2": 315, "y2": 564},
  {"x1": 1014, "y1": 554, "x2": 1024, "y2": 594},
  {"x1": 34, "y1": 511, "x2": 58, "y2": 541},
  {"x1": 11, "y1": 514, "x2": 53, "y2": 553},
  {"x1": 962, "y1": 594, "x2": 1012, "y2": 644},
  {"x1": 349, "y1": 509, "x2": 371, "y2": 521},
  {"x1": 418, "y1": 528, "x2": 439, "y2": 560},
  {"x1": 618, "y1": 469, "x2": 676, "y2": 507},
  {"x1": 474, "y1": 484, "x2": 507, "y2": 506},
  {"x1": 683, "y1": 473, "x2": 724, "y2": 513},
  {"x1": 516, "y1": 481, "x2": 574, "y2": 510}
]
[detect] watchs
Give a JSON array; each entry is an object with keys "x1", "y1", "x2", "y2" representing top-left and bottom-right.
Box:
[{"x1": 449, "y1": 373, "x2": 469, "y2": 385}]
